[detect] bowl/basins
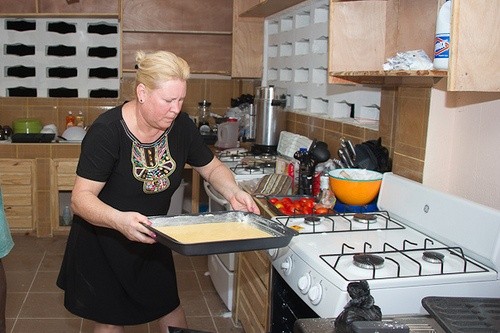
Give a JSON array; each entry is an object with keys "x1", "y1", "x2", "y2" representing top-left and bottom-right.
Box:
[
  {"x1": 41, "y1": 124, "x2": 87, "y2": 143},
  {"x1": 329, "y1": 168, "x2": 383, "y2": 207}
]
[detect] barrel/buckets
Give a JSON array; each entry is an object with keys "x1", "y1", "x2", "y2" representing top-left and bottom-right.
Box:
[{"x1": 252, "y1": 84, "x2": 288, "y2": 156}]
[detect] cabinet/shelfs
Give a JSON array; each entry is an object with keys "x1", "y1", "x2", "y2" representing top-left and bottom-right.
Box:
[
  {"x1": 0, "y1": 143, "x2": 200, "y2": 238},
  {"x1": 230, "y1": 0, "x2": 500, "y2": 132},
  {"x1": 232, "y1": 182, "x2": 273, "y2": 333}
]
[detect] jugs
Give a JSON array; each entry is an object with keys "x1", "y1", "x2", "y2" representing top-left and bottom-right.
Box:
[{"x1": 217, "y1": 118, "x2": 239, "y2": 147}]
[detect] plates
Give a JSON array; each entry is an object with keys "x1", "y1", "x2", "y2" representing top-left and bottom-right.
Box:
[{"x1": 13, "y1": 119, "x2": 41, "y2": 134}]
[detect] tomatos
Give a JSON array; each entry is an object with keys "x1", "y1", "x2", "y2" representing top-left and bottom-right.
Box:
[{"x1": 269, "y1": 197, "x2": 329, "y2": 216}]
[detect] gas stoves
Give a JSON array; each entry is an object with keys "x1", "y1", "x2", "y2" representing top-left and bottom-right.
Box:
[
  {"x1": 217, "y1": 131, "x2": 312, "y2": 182},
  {"x1": 268, "y1": 172, "x2": 499, "y2": 320}
]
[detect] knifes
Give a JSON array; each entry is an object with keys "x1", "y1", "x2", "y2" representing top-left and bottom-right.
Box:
[{"x1": 333, "y1": 137, "x2": 359, "y2": 167}]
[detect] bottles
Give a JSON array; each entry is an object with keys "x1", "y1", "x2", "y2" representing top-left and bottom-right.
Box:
[
  {"x1": 284, "y1": 147, "x2": 321, "y2": 200},
  {"x1": 76, "y1": 111, "x2": 84, "y2": 129},
  {"x1": 66, "y1": 110, "x2": 76, "y2": 129},
  {"x1": 433, "y1": 0, "x2": 450, "y2": 70}
]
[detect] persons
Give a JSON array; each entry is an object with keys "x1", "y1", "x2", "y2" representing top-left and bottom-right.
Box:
[{"x1": 56, "y1": 49, "x2": 260, "y2": 333}]
